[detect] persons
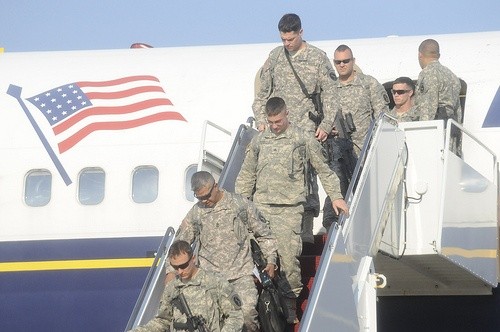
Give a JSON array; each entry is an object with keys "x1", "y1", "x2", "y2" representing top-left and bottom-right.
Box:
[
  {"x1": 233, "y1": 97, "x2": 350, "y2": 321},
  {"x1": 389, "y1": 77, "x2": 421, "y2": 121},
  {"x1": 322, "y1": 45, "x2": 391, "y2": 235},
  {"x1": 413, "y1": 38, "x2": 462, "y2": 158},
  {"x1": 126, "y1": 240, "x2": 245, "y2": 332},
  {"x1": 166, "y1": 170, "x2": 276, "y2": 331},
  {"x1": 251, "y1": 14, "x2": 342, "y2": 255}
]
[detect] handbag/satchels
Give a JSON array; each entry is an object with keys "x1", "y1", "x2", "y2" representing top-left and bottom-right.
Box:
[
  {"x1": 311, "y1": 93, "x2": 323, "y2": 110},
  {"x1": 305, "y1": 192, "x2": 321, "y2": 218},
  {"x1": 433, "y1": 105, "x2": 458, "y2": 127},
  {"x1": 256, "y1": 288, "x2": 285, "y2": 332}
]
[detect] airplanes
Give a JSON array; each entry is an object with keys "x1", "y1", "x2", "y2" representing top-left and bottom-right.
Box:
[{"x1": 0, "y1": 27, "x2": 500, "y2": 332}]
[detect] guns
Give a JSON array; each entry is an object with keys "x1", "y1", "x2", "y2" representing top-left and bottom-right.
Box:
[
  {"x1": 172, "y1": 292, "x2": 209, "y2": 332},
  {"x1": 309, "y1": 92, "x2": 329, "y2": 165},
  {"x1": 309, "y1": 165, "x2": 318, "y2": 196},
  {"x1": 250, "y1": 238, "x2": 271, "y2": 288},
  {"x1": 334, "y1": 108, "x2": 360, "y2": 189}
]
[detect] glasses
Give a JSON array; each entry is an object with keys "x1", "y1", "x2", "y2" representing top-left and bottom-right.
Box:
[
  {"x1": 170, "y1": 257, "x2": 192, "y2": 270},
  {"x1": 194, "y1": 186, "x2": 214, "y2": 200},
  {"x1": 334, "y1": 58, "x2": 350, "y2": 65},
  {"x1": 392, "y1": 89, "x2": 411, "y2": 95}
]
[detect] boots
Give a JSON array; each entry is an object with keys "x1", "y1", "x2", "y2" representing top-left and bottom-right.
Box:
[
  {"x1": 286, "y1": 297, "x2": 301, "y2": 324},
  {"x1": 301, "y1": 212, "x2": 315, "y2": 245}
]
